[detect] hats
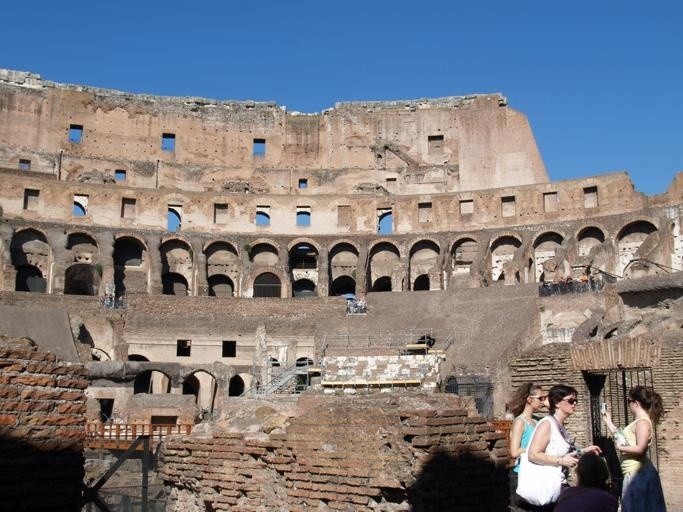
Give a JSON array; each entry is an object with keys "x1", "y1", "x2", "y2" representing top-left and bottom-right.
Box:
[{"x1": 579, "y1": 453, "x2": 609, "y2": 485}]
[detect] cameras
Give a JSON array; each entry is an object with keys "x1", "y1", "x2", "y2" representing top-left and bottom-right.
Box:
[{"x1": 602, "y1": 403, "x2": 608, "y2": 414}]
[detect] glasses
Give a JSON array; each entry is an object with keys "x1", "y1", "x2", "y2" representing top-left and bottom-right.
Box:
[
  {"x1": 628, "y1": 398, "x2": 637, "y2": 404},
  {"x1": 562, "y1": 397, "x2": 578, "y2": 405},
  {"x1": 529, "y1": 395, "x2": 546, "y2": 401}
]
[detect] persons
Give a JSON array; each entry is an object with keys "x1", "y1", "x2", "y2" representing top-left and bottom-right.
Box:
[
  {"x1": 543, "y1": 273, "x2": 588, "y2": 292},
  {"x1": 347, "y1": 292, "x2": 366, "y2": 313},
  {"x1": 510, "y1": 383, "x2": 602, "y2": 512},
  {"x1": 553, "y1": 453, "x2": 617, "y2": 512},
  {"x1": 601, "y1": 386, "x2": 666, "y2": 512}
]
